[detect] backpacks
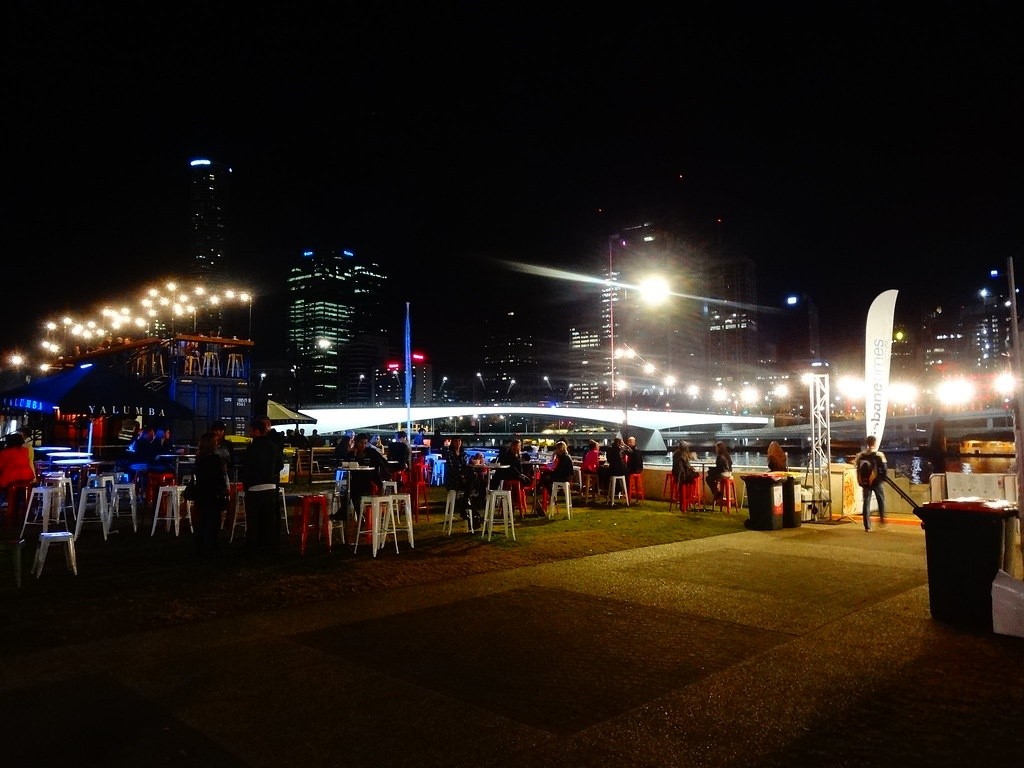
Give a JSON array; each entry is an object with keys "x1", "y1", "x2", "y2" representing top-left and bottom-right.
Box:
[{"x1": 857, "y1": 452, "x2": 879, "y2": 487}]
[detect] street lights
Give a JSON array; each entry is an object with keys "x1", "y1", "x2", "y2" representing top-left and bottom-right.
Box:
[{"x1": 609, "y1": 234, "x2": 620, "y2": 396}]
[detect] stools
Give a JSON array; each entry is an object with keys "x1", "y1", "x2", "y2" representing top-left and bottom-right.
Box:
[
  {"x1": 0, "y1": 453, "x2": 740, "y2": 587},
  {"x1": 225, "y1": 353, "x2": 245, "y2": 378},
  {"x1": 185, "y1": 356, "x2": 201, "y2": 375},
  {"x1": 202, "y1": 352, "x2": 222, "y2": 376}
]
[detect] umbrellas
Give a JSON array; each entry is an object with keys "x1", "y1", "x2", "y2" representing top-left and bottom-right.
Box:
[
  {"x1": 267, "y1": 399, "x2": 317, "y2": 426},
  {"x1": 0, "y1": 365, "x2": 187, "y2": 459}
]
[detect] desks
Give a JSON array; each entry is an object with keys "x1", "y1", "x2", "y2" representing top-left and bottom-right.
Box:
[
  {"x1": 33, "y1": 446, "x2": 71, "y2": 461},
  {"x1": 690, "y1": 461, "x2": 716, "y2": 512},
  {"x1": 52, "y1": 459, "x2": 94, "y2": 481},
  {"x1": 410, "y1": 444, "x2": 427, "y2": 457},
  {"x1": 173, "y1": 445, "x2": 198, "y2": 454},
  {"x1": 159, "y1": 454, "x2": 196, "y2": 483},
  {"x1": 336, "y1": 466, "x2": 375, "y2": 526},
  {"x1": 175, "y1": 335, "x2": 254, "y2": 352},
  {"x1": 519, "y1": 460, "x2": 550, "y2": 518},
  {"x1": 46, "y1": 452, "x2": 93, "y2": 465},
  {"x1": 469, "y1": 463, "x2": 510, "y2": 495}
]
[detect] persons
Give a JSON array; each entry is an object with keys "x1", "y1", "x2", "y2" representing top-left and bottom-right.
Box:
[
  {"x1": 114, "y1": 426, "x2": 189, "y2": 510},
  {"x1": 672, "y1": 441, "x2": 699, "y2": 484},
  {"x1": 855, "y1": 435, "x2": 887, "y2": 533},
  {"x1": 767, "y1": 441, "x2": 787, "y2": 472},
  {"x1": 330, "y1": 427, "x2": 426, "y2": 521},
  {"x1": 192, "y1": 416, "x2": 325, "y2": 558},
  {"x1": 580, "y1": 437, "x2": 643, "y2": 495},
  {"x1": 442, "y1": 438, "x2": 573, "y2": 521},
  {"x1": 706, "y1": 442, "x2": 732, "y2": 499},
  {"x1": 0, "y1": 428, "x2": 36, "y2": 489}
]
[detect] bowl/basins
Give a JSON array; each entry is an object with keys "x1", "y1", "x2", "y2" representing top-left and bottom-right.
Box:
[{"x1": 472, "y1": 459, "x2": 480, "y2": 466}]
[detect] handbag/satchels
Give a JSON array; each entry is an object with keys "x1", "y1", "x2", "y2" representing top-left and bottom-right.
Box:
[{"x1": 184, "y1": 464, "x2": 196, "y2": 500}]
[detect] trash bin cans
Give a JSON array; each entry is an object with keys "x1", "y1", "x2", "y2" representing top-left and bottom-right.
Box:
[
  {"x1": 915, "y1": 498, "x2": 1018, "y2": 631},
  {"x1": 785, "y1": 476, "x2": 804, "y2": 528},
  {"x1": 740, "y1": 473, "x2": 786, "y2": 532}
]
